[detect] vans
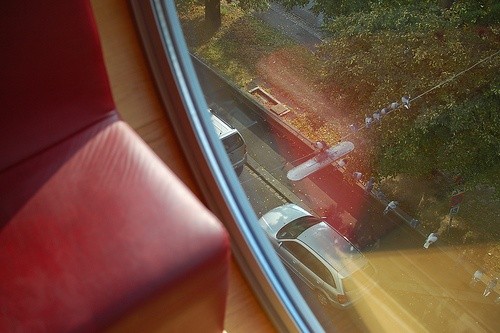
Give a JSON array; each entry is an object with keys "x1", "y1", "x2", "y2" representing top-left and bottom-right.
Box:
[{"x1": 208, "y1": 107, "x2": 248, "y2": 171}]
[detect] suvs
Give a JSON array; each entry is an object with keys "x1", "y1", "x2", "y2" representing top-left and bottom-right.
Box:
[{"x1": 258, "y1": 203, "x2": 381, "y2": 310}]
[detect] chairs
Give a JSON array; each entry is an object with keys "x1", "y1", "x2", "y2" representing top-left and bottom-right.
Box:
[{"x1": 0, "y1": 0, "x2": 232, "y2": 332}]
